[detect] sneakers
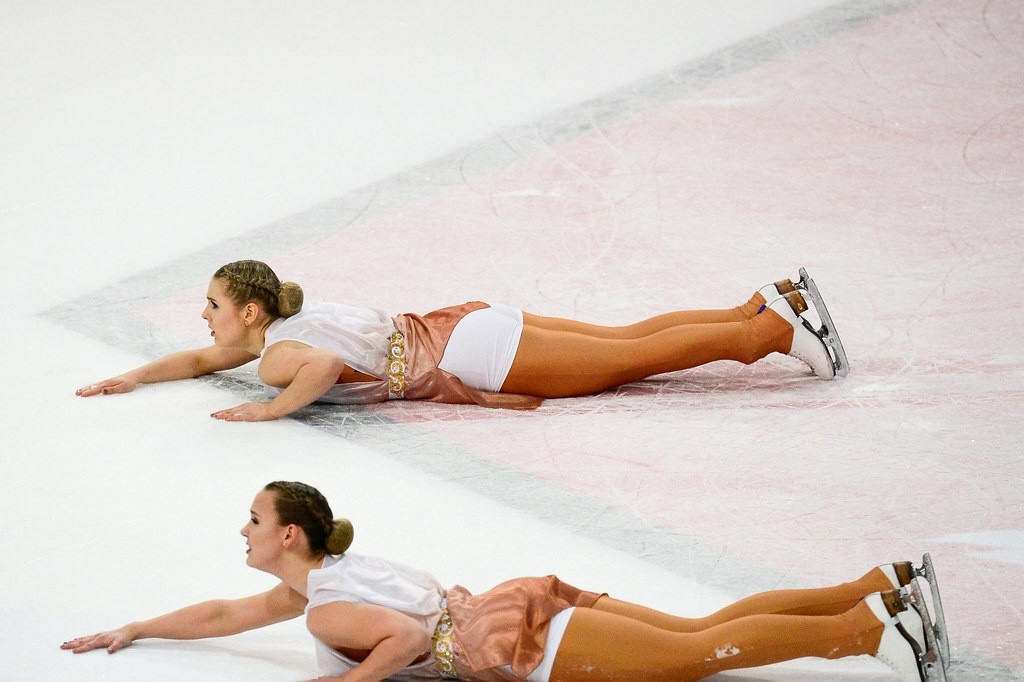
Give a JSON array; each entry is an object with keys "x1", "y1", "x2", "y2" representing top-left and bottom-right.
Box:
[
  {"x1": 759, "y1": 267, "x2": 851, "y2": 382},
  {"x1": 878, "y1": 553, "x2": 951, "y2": 671},
  {"x1": 865, "y1": 578, "x2": 947, "y2": 682}
]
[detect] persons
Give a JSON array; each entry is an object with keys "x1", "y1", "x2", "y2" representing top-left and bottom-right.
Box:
[
  {"x1": 78, "y1": 260, "x2": 848, "y2": 419},
  {"x1": 61, "y1": 481, "x2": 951, "y2": 682}
]
